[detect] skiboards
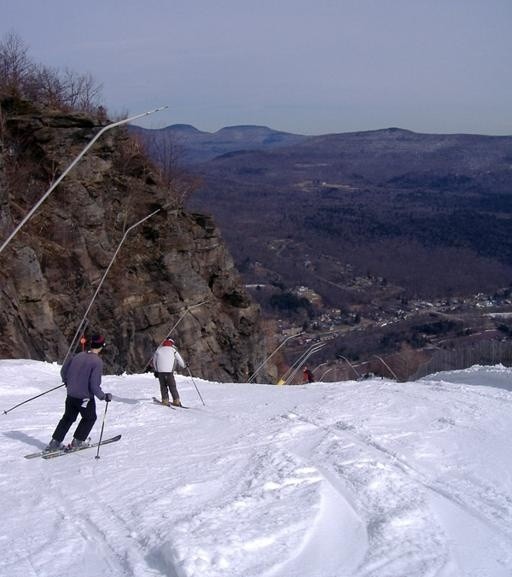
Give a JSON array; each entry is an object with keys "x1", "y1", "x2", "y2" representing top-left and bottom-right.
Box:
[
  {"x1": 152, "y1": 397, "x2": 189, "y2": 410},
  {"x1": 23, "y1": 434, "x2": 121, "y2": 459}
]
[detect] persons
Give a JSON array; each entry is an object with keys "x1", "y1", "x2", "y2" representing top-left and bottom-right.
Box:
[
  {"x1": 152, "y1": 338, "x2": 190, "y2": 406},
  {"x1": 48, "y1": 333, "x2": 113, "y2": 450},
  {"x1": 303, "y1": 364, "x2": 314, "y2": 384}
]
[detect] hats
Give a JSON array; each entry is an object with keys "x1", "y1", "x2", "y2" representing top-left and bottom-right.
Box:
[
  {"x1": 163, "y1": 336, "x2": 175, "y2": 346},
  {"x1": 91, "y1": 332, "x2": 106, "y2": 348}
]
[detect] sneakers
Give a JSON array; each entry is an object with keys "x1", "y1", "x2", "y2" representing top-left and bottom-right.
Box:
[
  {"x1": 173, "y1": 400, "x2": 182, "y2": 406},
  {"x1": 163, "y1": 400, "x2": 169, "y2": 405},
  {"x1": 72, "y1": 438, "x2": 85, "y2": 447},
  {"x1": 49, "y1": 439, "x2": 64, "y2": 450}
]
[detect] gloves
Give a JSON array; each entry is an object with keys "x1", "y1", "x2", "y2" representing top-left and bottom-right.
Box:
[
  {"x1": 186, "y1": 362, "x2": 190, "y2": 368},
  {"x1": 154, "y1": 372, "x2": 159, "y2": 377},
  {"x1": 103, "y1": 393, "x2": 112, "y2": 402}
]
